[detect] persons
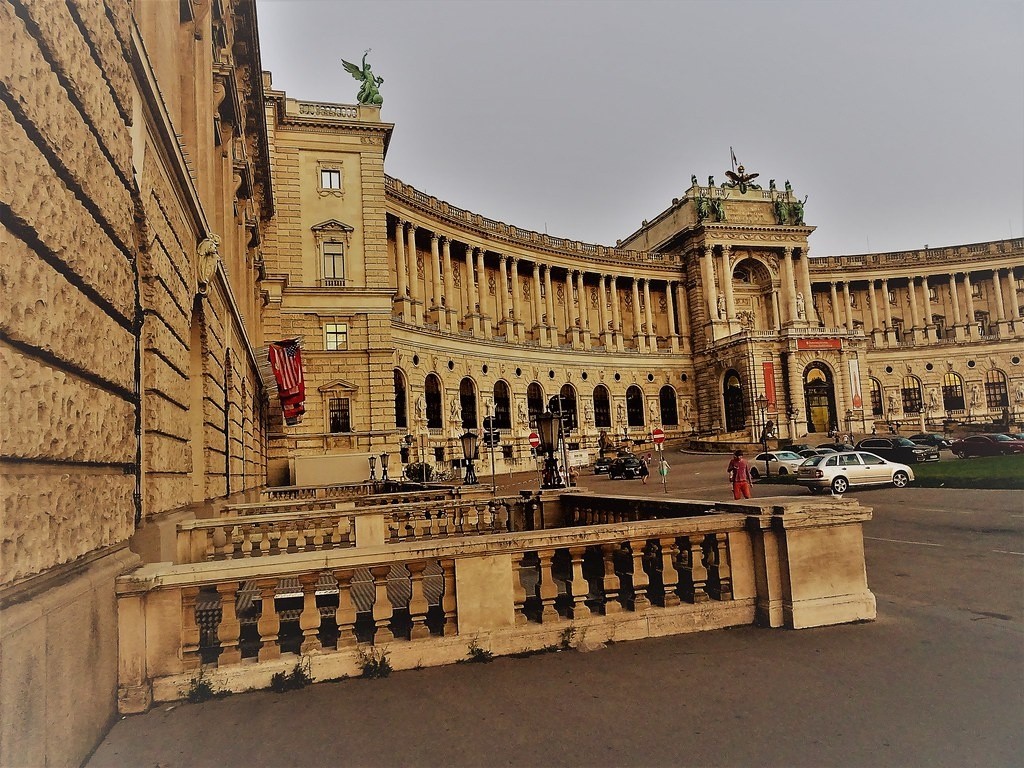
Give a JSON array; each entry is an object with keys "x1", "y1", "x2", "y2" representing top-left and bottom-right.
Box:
[
  {"x1": 486, "y1": 398, "x2": 498, "y2": 417},
  {"x1": 584, "y1": 399, "x2": 594, "y2": 420},
  {"x1": 517, "y1": 400, "x2": 529, "y2": 421},
  {"x1": 417, "y1": 393, "x2": 427, "y2": 417},
  {"x1": 727, "y1": 450, "x2": 753, "y2": 499},
  {"x1": 772, "y1": 195, "x2": 809, "y2": 226},
  {"x1": 638, "y1": 456, "x2": 650, "y2": 485},
  {"x1": 617, "y1": 400, "x2": 627, "y2": 420},
  {"x1": 649, "y1": 401, "x2": 658, "y2": 422},
  {"x1": 683, "y1": 400, "x2": 693, "y2": 418},
  {"x1": 717, "y1": 290, "x2": 726, "y2": 311},
  {"x1": 559, "y1": 465, "x2": 580, "y2": 486},
  {"x1": 1014, "y1": 380, "x2": 1024, "y2": 400},
  {"x1": 342, "y1": 53, "x2": 381, "y2": 104},
  {"x1": 828, "y1": 421, "x2": 855, "y2": 446},
  {"x1": 657, "y1": 457, "x2": 671, "y2": 484},
  {"x1": 929, "y1": 388, "x2": 941, "y2": 406},
  {"x1": 871, "y1": 420, "x2": 901, "y2": 435},
  {"x1": 971, "y1": 384, "x2": 982, "y2": 403},
  {"x1": 450, "y1": 396, "x2": 463, "y2": 420},
  {"x1": 797, "y1": 291, "x2": 805, "y2": 312},
  {"x1": 694, "y1": 190, "x2": 731, "y2": 221},
  {"x1": 889, "y1": 390, "x2": 898, "y2": 408}
]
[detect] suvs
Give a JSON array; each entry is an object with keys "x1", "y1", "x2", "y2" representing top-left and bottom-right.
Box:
[
  {"x1": 852, "y1": 437, "x2": 941, "y2": 465},
  {"x1": 908, "y1": 433, "x2": 955, "y2": 450}
]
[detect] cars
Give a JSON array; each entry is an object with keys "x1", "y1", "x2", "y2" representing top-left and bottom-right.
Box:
[
  {"x1": 797, "y1": 451, "x2": 915, "y2": 493},
  {"x1": 593, "y1": 457, "x2": 616, "y2": 474},
  {"x1": 951, "y1": 433, "x2": 1024, "y2": 459},
  {"x1": 799, "y1": 449, "x2": 850, "y2": 462},
  {"x1": 607, "y1": 457, "x2": 649, "y2": 481},
  {"x1": 747, "y1": 450, "x2": 814, "y2": 478}
]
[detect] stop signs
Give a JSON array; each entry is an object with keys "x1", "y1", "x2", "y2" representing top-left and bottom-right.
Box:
[
  {"x1": 653, "y1": 428, "x2": 665, "y2": 444},
  {"x1": 528, "y1": 433, "x2": 540, "y2": 447}
]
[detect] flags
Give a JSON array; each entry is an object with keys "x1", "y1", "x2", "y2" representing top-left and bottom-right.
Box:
[
  {"x1": 267, "y1": 337, "x2": 306, "y2": 425},
  {"x1": 731, "y1": 150, "x2": 737, "y2": 165}
]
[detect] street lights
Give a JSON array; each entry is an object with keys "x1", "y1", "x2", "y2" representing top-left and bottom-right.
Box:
[{"x1": 754, "y1": 392, "x2": 771, "y2": 478}]
